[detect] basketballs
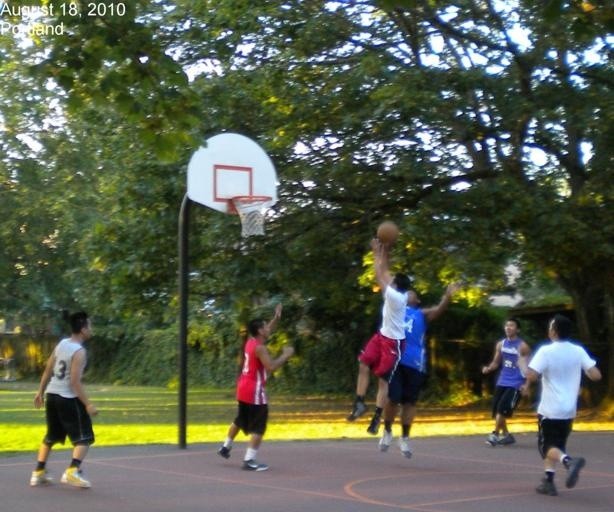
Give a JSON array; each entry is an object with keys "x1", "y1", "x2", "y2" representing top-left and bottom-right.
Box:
[{"x1": 377, "y1": 222, "x2": 399, "y2": 243}]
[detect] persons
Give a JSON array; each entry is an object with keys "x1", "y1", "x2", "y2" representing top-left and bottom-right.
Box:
[
  {"x1": 344, "y1": 238, "x2": 410, "y2": 435},
  {"x1": 376, "y1": 283, "x2": 461, "y2": 459},
  {"x1": 520, "y1": 314, "x2": 602, "y2": 497},
  {"x1": 29, "y1": 310, "x2": 98, "y2": 489},
  {"x1": 482, "y1": 318, "x2": 531, "y2": 447},
  {"x1": 217, "y1": 302, "x2": 295, "y2": 472}
]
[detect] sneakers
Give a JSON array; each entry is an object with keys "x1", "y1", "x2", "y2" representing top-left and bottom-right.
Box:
[
  {"x1": 536, "y1": 481, "x2": 558, "y2": 496},
  {"x1": 29, "y1": 469, "x2": 52, "y2": 487},
  {"x1": 217, "y1": 445, "x2": 231, "y2": 459},
  {"x1": 486, "y1": 433, "x2": 498, "y2": 448},
  {"x1": 566, "y1": 457, "x2": 586, "y2": 489},
  {"x1": 498, "y1": 433, "x2": 515, "y2": 446},
  {"x1": 242, "y1": 459, "x2": 270, "y2": 472},
  {"x1": 348, "y1": 397, "x2": 413, "y2": 460},
  {"x1": 59, "y1": 467, "x2": 91, "y2": 488}
]
